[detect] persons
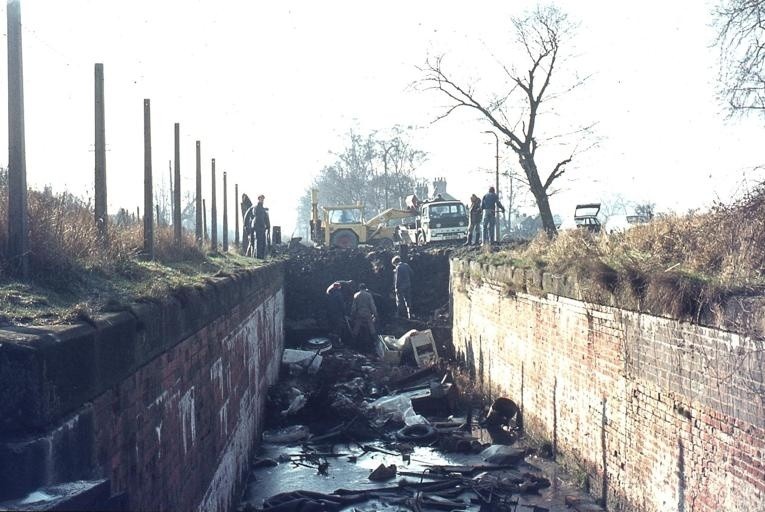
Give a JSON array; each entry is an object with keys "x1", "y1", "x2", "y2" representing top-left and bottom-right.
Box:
[
  {"x1": 392, "y1": 256, "x2": 415, "y2": 319},
  {"x1": 244, "y1": 194, "x2": 270, "y2": 259},
  {"x1": 431, "y1": 194, "x2": 445, "y2": 202},
  {"x1": 325, "y1": 279, "x2": 379, "y2": 339},
  {"x1": 463, "y1": 187, "x2": 505, "y2": 246}
]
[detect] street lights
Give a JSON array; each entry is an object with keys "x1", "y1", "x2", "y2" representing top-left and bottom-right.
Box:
[{"x1": 481, "y1": 130, "x2": 501, "y2": 241}]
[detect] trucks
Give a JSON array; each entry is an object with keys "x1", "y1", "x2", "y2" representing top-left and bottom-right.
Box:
[{"x1": 398, "y1": 201, "x2": 469, "y2": 245}]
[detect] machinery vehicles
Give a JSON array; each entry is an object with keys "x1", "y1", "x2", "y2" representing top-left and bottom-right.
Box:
[{"x1": 309, "y1": 189, "x2": 422, "y2": 247}]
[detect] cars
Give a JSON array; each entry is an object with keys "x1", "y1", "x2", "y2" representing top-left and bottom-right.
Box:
[{"x1": 559, "y1": 201, "x2": 652, "y2": 233}]
